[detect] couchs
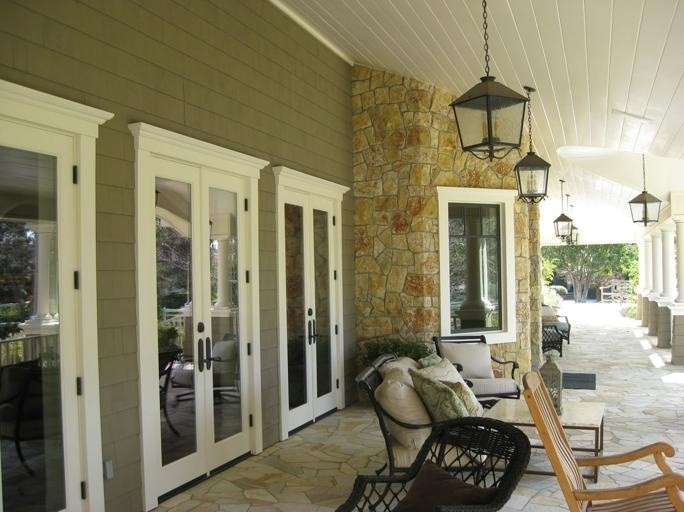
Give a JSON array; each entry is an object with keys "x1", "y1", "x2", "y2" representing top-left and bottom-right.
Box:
[
  {"x1": 355, "y1": 351, "x2": 487, "y2": 487},
  {"x1": 0, "y1": 344, "x2": 183, "y2": 475}
]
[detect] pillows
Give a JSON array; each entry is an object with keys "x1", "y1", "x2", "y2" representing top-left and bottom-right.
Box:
[
  {"x1": 212, "y1": 340, "x2": 235, "y2": 387},
  {"x1": 542, "y1": 307, "x2": 557, "y2": 322},
  {"x1": 438, "y1": 343, "x2": 494, "y2": 378},
  {"x1": 393, "y1": 458, "x2": 497, "y2": 512},
  {"x1": 374, "y1": 352, "x2": 483, "y2": 450}
]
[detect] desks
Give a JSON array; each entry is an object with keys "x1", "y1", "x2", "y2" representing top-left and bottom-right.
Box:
[{"x1": 482, "y1": 398, "x2": 606, "y2": 484}]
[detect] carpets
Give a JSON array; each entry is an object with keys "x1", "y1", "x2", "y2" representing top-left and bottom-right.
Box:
[{"x1": 562, "y1": 373, "x2": 596, "y2": 389}]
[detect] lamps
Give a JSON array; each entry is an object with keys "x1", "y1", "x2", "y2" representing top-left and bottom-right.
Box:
[
  {"x1": 628, "y1": 153, "x2": 663, "y2": 226},
  {"x1": 448, "y1": 0, "x2": 530, "y2": 161},
  {"x1": 513, "y1": 86, "x2": 552, "y2": 205},
  {"x1": 553, "y1": 180, "x2": 579, "y2": 249}
]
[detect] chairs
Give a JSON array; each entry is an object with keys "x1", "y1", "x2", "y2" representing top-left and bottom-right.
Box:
[
  {"x1": 542, "y1": 305, "x2": 570, "y2": 344},
  {"x1": 169, "y1": 333, "x2": 241, "y2": 407},
  {"x1": 335, "y1": 417, "x2": 531, "y2": 512},
  {"x1": 542, "y1": 323, "x2": 562, "y2": 357},
  {"x1": 599, "y1": 278, "x2": 630, "y2": 307},
  {"x1": 522, "y1": 369, "x2": 684, "y2": 512},
  {"x1": 432, "y1": 335, "x2": 520, "y2": 409}
]
